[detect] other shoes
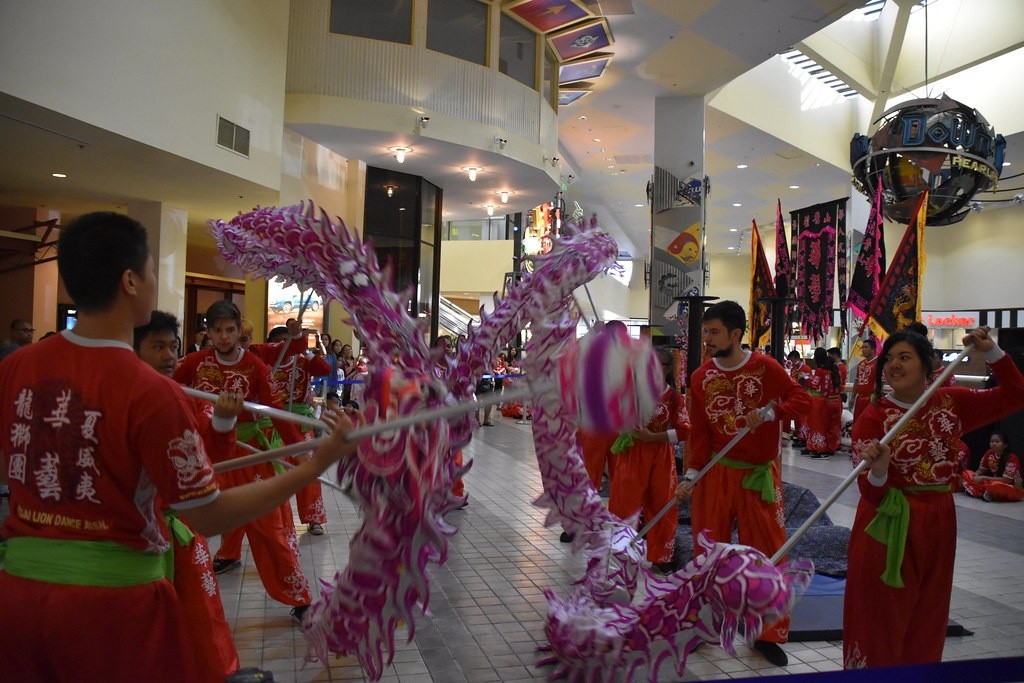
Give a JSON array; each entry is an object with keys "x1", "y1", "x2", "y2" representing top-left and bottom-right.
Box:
[
  {"x1": 983, "y1": 492, "x2": 992, "y2": 501},
  {"x1": 290, "y1": 604, "x2": 312, "y2": 623},
  {"x1": 482, "y1": 422, "x2": 495, "y2": 426},
  {"x1": 224, "y1": 666, "x2": 272, "y2": 683},
  {"x1": 309, "y1": 523, "x2": 324, "y2": 535},
  {"x1": 752, "y1": 640, "x2": 788, "y2": 666},
  {"x1": 212, "y1": 558, "x2": 240, "y2": 574},
  {"x1": 792, "y1": 441, "x2": 829, "y2": 459}
]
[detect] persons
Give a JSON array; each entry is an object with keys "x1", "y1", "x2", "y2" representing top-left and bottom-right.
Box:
[
  {"x1": 559, "y1": 320, "x2": 689, "y2": 576},
  {"x1": 674, "y1": 301, "x2": 813, "y2": 666},
  {"x1": 849, "y1": 339, "x2": 880, "y2": 460},
  {"x1": 950, "y1": 438, "x2": 970, "y2": 492},
  {"x1": 741, "y1": 343, "x2": 847, "y2": 460},
  {"x1": 843, "y1": 326, "x2": 1024, "y2": 670},
  {"x1": 0, "y1": 210, "x2": 371, "y2": 683},
  {"x1": 929, "y1": 349, "x2": 956, "y2": 388},
  {"x1": 431, "y1": 334, "x2": 517, "y2": 505},
  {"x1": 960, "y1": 431, "x2": 1024, "y2": 502}
]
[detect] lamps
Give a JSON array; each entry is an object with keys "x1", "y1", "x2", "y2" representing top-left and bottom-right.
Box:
[
  {"x1": 543, "y1": 156, "x2": 559, "y2": 167},
  {"x1": 971, "y1": 202, "x2": 984, "y2": 214},
  {"x1": 1014, "y1": 193, "x2": 1024, "y2": 206},
  {"x1": 495, "y1": 136, "x2": 507, "y2": 149},
  {"x1": 417, "y1": 117, "x2": 430, "y2": 128},
  {"x1": 568, "y1": 175, "x2": 574, "y2": 184}
]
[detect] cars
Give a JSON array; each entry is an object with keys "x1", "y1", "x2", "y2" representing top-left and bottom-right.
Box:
[{"x1": 268, "y1": 279, "x2": 324, "y2": 314}]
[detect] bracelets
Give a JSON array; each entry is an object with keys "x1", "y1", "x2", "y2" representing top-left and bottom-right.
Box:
[
  {"x1": 684, "y1": 477, "x2": 697, "y2": 486},
  {"x1": 318, "y1": 342, "x2": 323, "y2": 345}
]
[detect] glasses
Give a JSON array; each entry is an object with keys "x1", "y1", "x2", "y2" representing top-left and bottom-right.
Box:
[{"x1": 16, "y1": 327, "x2": 36, "y2": 334}]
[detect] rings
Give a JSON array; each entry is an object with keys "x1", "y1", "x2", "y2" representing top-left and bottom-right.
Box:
[{"x1": 230, "y1": 395, "x2": 236, "y2": 399}]
